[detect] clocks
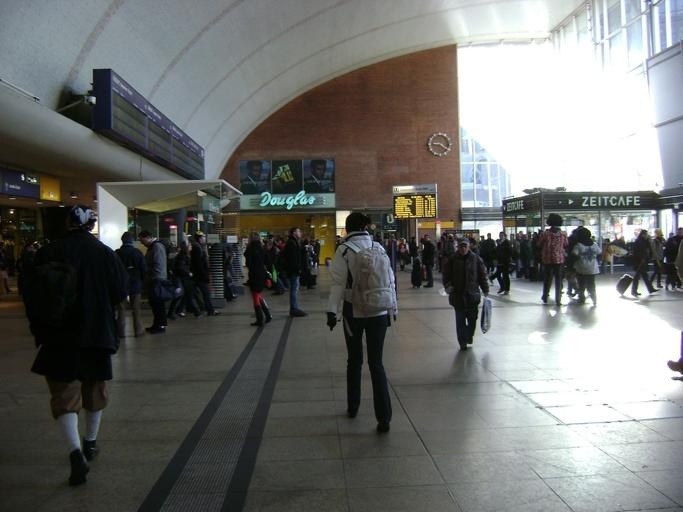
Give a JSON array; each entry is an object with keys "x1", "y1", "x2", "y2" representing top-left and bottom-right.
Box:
[{"x1": 428, "y1": 132, "x2": 452, "y2": 157}]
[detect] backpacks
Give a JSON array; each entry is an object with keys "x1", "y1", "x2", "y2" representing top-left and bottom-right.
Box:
[{"x1": 341, "y1": 240, "x2": 394, "y2": 314}]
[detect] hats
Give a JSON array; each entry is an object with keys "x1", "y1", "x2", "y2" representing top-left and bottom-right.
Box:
[
  {"x1": 455, "y1": 237, "x2": 470, "y2": 247},
  {"x1": 194, "y1": 230, "x2": 207, "y2": 240}
]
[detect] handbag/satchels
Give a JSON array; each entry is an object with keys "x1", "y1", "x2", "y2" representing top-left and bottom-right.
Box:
[
  {"x1": 148, "y1": 277, "x2": 173, "y2": 302},
  {"x1": 480, "y1": 296, "x2": 492, "y2": 334}
]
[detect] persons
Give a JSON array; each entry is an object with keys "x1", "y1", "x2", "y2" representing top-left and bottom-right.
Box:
[
  {"x1": 442, "y1": 237, "x2": 490, "y2": 349},
  {"x1": 334, "y1": 213, "x2": 682, "y2": 306},
  {"x1": 325, "y1": 212, "x2": 398, "y2": 432},
  {"x1": 18, "y1": 204, "x2": 129, "y2": 487},
  {"x1": 241, "y1": 161, "x2": 261, "y2": 194},
  {"x1": 0, "y1": 227, "x2": 320, "y2": 338},
  {"x1": 304, "y1": 160, "x2": 333, "y2": 192},
  {"x1": 667, "y1": 332, "x2": 683, "y2": 375}
]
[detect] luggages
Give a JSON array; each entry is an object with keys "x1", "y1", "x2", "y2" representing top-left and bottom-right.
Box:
[{"x1": 615, "y1": 273, "x2": 633, "y2": 296}]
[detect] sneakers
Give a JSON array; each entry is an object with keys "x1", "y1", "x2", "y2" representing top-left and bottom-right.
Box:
[
  {"x1": 631, "y1": 282, "x2": 683, "y2": 296},
  {"x1": 68, "y1": 453, "x2": 90, "y2": 487},
  {"x1": 145, "y1": 325, "x2": 166, "y2": 334},
  {"x1": 82, "y1": 446, "x2": 101, "y2": 462},
  {"x1": 289, "y1": 309, "x2": 307, "y2": 317},
  {"x1": 667, "y1": 358, "x2": 683, "y2": 375},
  {"x1": 459, "y1": 337, "x2": 473, "y2": 352},
  {"x1": 497, "y1": 287, "x2": 511, "y2": 295},
  {"x1": 376, "y1": 423, "x2": 390, "y2": 433},
  {"x1": 250, "y1": 314, "x2": 273, "y2": 326},
  {"x1": 167, "y1": 308, "x2": 225, "y2": 320},
  {"x1": 347, "y1": 407, "x2": 357, "y2": 419},
  {"x1": 542, "y1": 296, "x2": 563, "y2": 306}
]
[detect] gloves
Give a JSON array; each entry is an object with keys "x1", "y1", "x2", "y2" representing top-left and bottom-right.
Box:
[{"x1": 326, "y1": 312, "x2": 338, "y2": 331}]
